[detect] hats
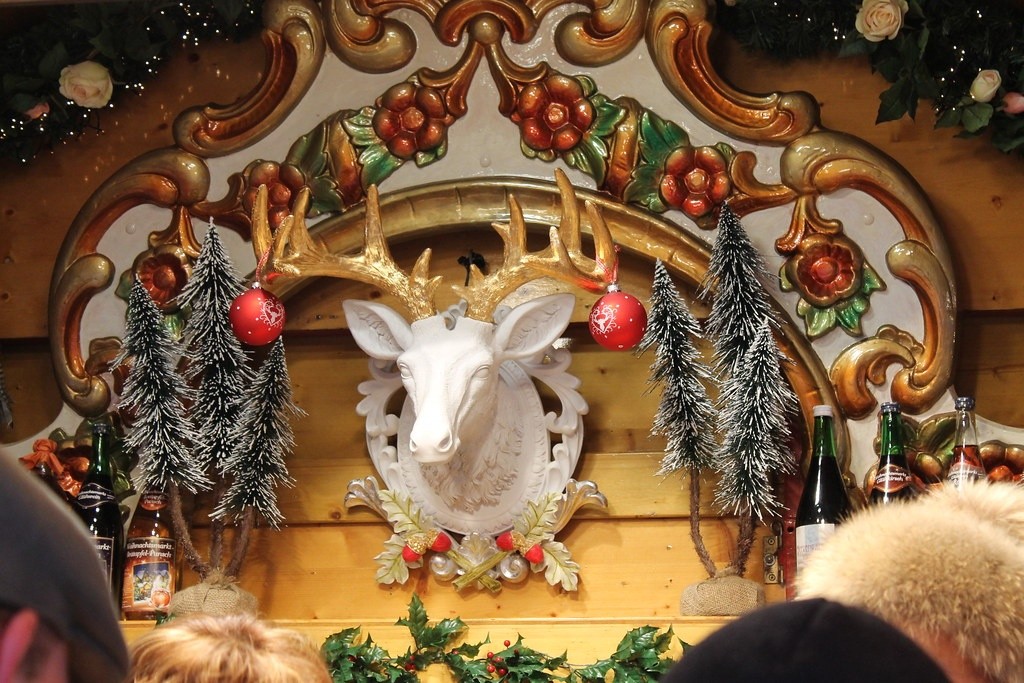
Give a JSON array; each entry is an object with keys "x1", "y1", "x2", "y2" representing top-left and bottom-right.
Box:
[
  {"x1": 660, "y1": 597, "x2": 952, "y2": 683},
  {"x1": 0, "y1": 446, "x2": 129, "y2": 683},
  {"x1": 794, "y1": 476, "x2": 1024, "y2": 683}
]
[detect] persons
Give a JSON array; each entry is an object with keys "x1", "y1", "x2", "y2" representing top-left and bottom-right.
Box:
[
  {"x1": 655, "y1": 480, "x2": 1024, "y2": 683},
  {"x1": 0, "y1": 608, "x2": 333, "y2": 681}
]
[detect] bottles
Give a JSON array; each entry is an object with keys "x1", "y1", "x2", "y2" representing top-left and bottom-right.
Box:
[
  {"x1": 867, "y1": 401, "x2": 915, "y2": 509},
  {"x1": 75, "y1": 424, "x2": 124, "y2": 618},
  {"x1": 33, "y1": 438, "x2": 58, "y2": 488},
  {"x1": 947, "y1": 397, "x2": 988, "y2": 487},
  {"x1": 795, "y1": 405, "x2": 852, "y2": 597},
  {"x1": 122, "y1": 487, "x2": 176, "y2": 619}
]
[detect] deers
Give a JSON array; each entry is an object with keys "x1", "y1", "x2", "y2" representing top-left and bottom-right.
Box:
[{"x1": 250, "y1": 167, "x2": 622, "y2": 514}]
